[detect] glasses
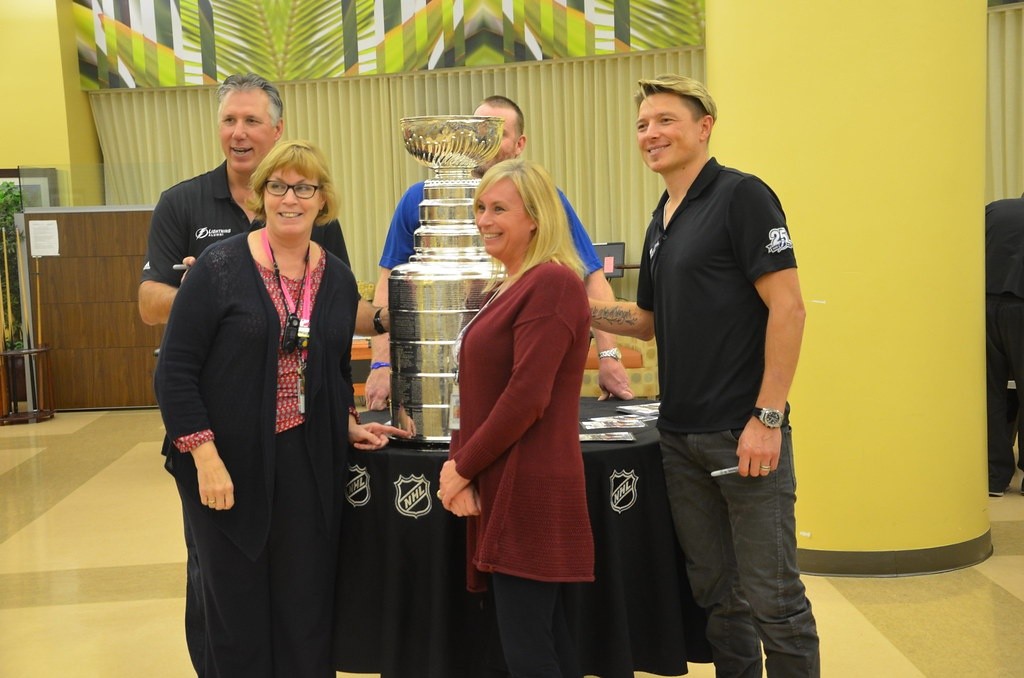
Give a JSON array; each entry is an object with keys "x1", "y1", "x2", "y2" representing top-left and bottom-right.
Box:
[{"x1": 264, "y1": 181, "x2": 324, "y2": 199}]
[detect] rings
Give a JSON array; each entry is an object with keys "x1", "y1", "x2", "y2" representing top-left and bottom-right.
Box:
[{"x1": 761, "y1": 466, "x2": 771, "y2": 471}]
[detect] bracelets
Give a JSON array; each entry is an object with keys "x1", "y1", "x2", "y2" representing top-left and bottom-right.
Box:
[
  {"x1": 371, "y1": 362, "x2": 391, "y2": 369},
  {"x1": 373, "y1": 307, "x2": 388, "y2": 334}
]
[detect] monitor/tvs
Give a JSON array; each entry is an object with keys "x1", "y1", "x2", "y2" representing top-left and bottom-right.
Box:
[{"x1": 592, "y1": 242, "x2": 625, "y2": 278}]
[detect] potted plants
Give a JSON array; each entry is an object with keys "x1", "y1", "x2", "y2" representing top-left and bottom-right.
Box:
[{"x1": 0, "y1": 182, "x2": 33, "y2": 403}]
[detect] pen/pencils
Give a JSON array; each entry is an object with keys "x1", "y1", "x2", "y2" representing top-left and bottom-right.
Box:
[{"x1": 708, "y1": 465, "x2": 741, "y2": 479}]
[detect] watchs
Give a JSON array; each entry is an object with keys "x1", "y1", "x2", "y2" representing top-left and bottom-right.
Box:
[
  {"x1": 752, "y1": 406, "x2": 783, "y2": 429},
  {"x1": 599, "y1": 348, "x2": 622, "y2": 360}
]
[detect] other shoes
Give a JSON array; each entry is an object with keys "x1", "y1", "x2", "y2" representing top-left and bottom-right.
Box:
[{"x1": 988, "y1": 480, "x2": 1014, "y2": 496}]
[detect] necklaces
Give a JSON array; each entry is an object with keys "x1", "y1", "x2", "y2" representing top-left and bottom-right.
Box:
[{"x1": 663, "y1": 198, "x2": 671, "y2": 230}]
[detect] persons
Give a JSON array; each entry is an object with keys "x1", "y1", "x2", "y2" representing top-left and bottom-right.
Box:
[
  {"x1": 439, "y1": 159, "x2": 595, "y2": 678},
  {"x1": 985, "y1": 192, "x2": 1024, "y2": 497},
  {"x1": 365, "y1": 95, "x2": 635, "y2": 410},
  {"x1": 155, "y1": 140, "x2": 410, "y2": 678},
  {"x1": 586, "y1": 74, "x2": 822, "y2": 678},
  {"x1": 139, "y1": 74, "x2": 394, "y2": 337}
]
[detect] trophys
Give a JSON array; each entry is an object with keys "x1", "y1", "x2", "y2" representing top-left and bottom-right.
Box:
[{"x1": 391, "y1": 114, "x2": 510, "y2": 434}]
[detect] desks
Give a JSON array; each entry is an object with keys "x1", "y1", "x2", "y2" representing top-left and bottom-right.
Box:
[{"x1": 330, "y1": 397, "x2": 713, "y2": 678}]
